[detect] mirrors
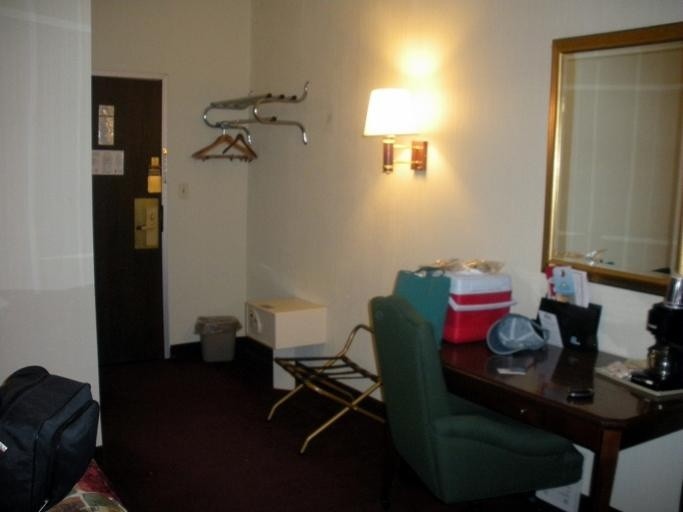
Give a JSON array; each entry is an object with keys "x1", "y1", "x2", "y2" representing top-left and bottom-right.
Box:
[{"x1": 541, "y1": 20, "x2": 682, "y2": 293}]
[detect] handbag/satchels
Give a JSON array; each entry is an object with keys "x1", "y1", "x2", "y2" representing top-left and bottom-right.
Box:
[
  {"x1": 1, "y1": 366, "x2": 98, "y2": 512},
  {"x1": 393, "y1": 267, "x2": 450, "y2": 351}
]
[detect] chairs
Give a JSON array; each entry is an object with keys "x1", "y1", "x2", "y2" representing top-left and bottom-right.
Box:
[
  {"x1": 368, "y1": 295, "x2": 584, "y2": 512},
  {"x1": 267, "y1": 325, "x2": 387, "y2": 455}
]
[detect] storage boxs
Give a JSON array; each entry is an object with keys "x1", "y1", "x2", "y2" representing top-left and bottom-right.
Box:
[{"x1": 412, "y1": 266, "x2": 517, "y2": 345}]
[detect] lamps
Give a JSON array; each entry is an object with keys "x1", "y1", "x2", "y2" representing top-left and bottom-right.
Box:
[{"x1": 363, "y1": 89, "x2": 427, "y2": 172}]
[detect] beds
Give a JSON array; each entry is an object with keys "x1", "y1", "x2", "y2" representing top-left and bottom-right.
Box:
[{"x1": 42, "y1": 461, "x2": 128, "y2": 511}]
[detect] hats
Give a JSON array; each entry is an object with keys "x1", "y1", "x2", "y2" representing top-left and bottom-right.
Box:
[{"x1": 486, "y1": 314, "x2": 549, "y2": 355}]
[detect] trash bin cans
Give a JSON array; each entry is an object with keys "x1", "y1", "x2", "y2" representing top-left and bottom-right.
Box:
[{"x1": 196, "y1": 316, "x2": 236, "y2": 362}]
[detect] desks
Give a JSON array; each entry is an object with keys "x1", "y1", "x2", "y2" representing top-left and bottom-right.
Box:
[
  {"x1": 245, "y1": 299, "x2": 326, "y2": 391},
  {"x1": 437, "y1": 331, "x2": 683, "y2": 511}
]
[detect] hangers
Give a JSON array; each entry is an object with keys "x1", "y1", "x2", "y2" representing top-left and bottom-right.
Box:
[
  {"x1": 223, "y1": 121, "x2": 259, "y2": 158},
  {"x1": 192, "y1": 121, "x2": 255, "y2": 162}
]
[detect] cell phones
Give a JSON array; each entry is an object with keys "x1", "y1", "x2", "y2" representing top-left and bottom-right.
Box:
[{"x1": 567, "y1": 389, "x2": 594, "y2": 401}]
